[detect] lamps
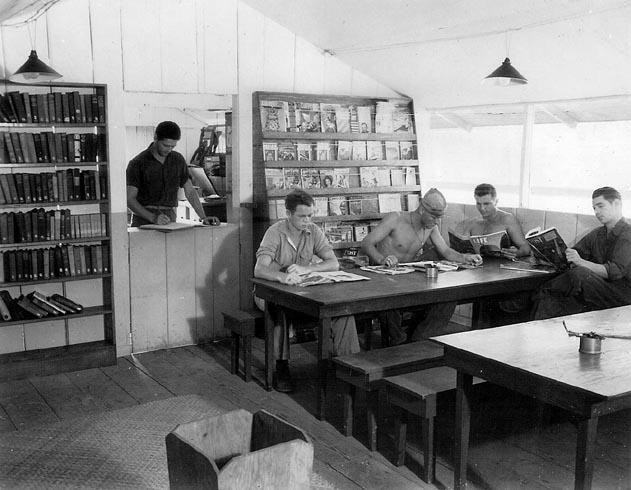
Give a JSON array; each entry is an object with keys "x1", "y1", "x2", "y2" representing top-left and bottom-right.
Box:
[
  {"x1": 478, "y1": 30, "x2": 529, "y2": 90},
  {"x1": 6, "y1": 16, "x2": 62, "y2": 83}
]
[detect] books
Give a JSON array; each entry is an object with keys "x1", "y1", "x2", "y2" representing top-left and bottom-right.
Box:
[
  {"x1": 501, "y1": 258, "x2": 557, "y2": 275},
  {"x1": 259, "y1": 97, "x2": 422, "y2": 262},
  {"x1": 300, "y1": 269, "x2": 370, "y2": 288},
  {"x1": 446, "y1": 226, "x2": 511, "y2": 260},
  {"x1": 525, "y1": 226, "x2": 575, "y2": 274},
  {"x1": 140, "y1": 218, "x2": 207, "y2": 233},
  {"x1": 0, "y1": 90, "x2": 110, "y2": 322},
  {"x1": 364, "y1": 259, "x2": 482, "y2": 276}
]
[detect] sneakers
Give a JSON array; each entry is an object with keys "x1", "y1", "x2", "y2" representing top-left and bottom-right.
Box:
[{"x1": 274, "y1": 367, "x2": 294, "y2": 393}]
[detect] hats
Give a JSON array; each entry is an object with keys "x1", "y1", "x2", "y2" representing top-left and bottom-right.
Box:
[{"x1": 423, "y1": 187, "x2": 447, "y2": 217}]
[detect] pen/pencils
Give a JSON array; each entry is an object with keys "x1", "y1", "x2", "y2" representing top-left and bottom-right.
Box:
[{"x1": 156, "y1": 207, "x2": 164, "y2": 214}]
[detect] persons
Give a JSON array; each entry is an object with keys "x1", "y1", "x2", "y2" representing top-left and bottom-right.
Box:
[
  {"x1": 498, "y1": 186, "x2": 631, "y2": 321},
  {"x1": 454, "y1": 182, "x2": 534, "y2": 260},
  {"x1": 126, "y1": 119, "x2": 222, "y2": 228},
  {"x1": 360, "y1": 188, "x2": 488, "y2": 345},
  {"x1": 253, "y1": 188, "x2": 363, "y2": 393}
]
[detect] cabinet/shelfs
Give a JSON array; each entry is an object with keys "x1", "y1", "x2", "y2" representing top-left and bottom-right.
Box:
[
  {"x1": 252, "y1": 90, "x2": 422, "y2": 268},
  {"x1": 0, "y1": 78, "x2": 118, "y2": 383}
]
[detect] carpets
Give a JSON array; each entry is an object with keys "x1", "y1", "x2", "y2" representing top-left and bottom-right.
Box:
[{"x1": 0, "y1": 392, "x2": 338, "y2": 490}]
